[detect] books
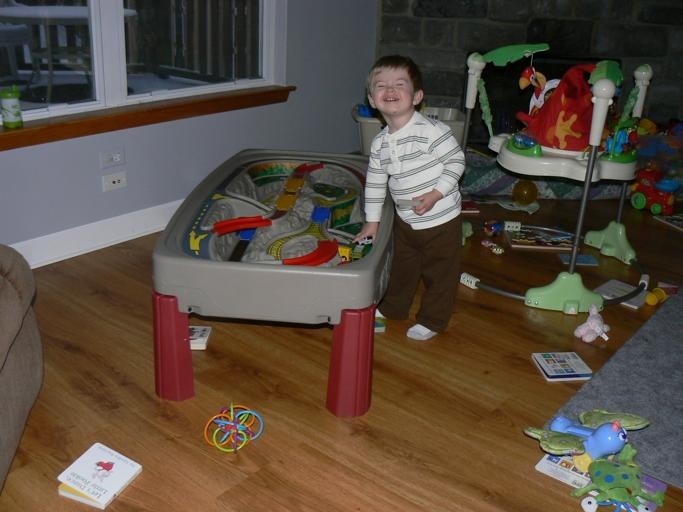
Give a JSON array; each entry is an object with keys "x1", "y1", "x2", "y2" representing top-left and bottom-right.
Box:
[
  {"x1": 559, "y1": 253, "x2": 599, "y2": 266},
  {"x1": 593, "y1": 279, "x2": 652, "y2": 310},
  {"x1": 188, "y1": 325, "x2": 212, "y2": 350},
  {"x1": 534, "y1": 351, "x2": 593, "y2": 382},
  {"x1": 57, "y1": 441, "x2": 143, "y2": 510},
  {"x1": 504, "y1": 225, "x2": 573, "y2": 250}
]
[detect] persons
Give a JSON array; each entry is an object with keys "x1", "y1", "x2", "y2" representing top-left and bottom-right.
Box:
[{"x1": 351, "y1": 54, "x2": 468, "y2": 342}]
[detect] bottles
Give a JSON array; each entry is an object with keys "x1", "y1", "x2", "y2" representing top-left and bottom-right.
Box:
[{"x1": 0, "y1": 89, "x2": 23, "y2": 129}]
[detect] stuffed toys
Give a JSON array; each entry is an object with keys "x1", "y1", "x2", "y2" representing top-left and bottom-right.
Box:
[{"x1": 573, "y1": 303, "x2": 610, "y2": 344}]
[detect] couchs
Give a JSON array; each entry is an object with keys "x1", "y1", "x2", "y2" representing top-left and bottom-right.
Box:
[{"x1": 1, "y1": 243, "x2": 45, "y2": 495}]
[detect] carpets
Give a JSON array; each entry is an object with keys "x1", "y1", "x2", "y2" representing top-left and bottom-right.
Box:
[{"x1": 538, "y1": 286, "x2": 683, "y2": 490}]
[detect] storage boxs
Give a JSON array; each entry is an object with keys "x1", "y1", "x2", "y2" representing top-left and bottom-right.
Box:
[{"x1": 351, "y1": 104, "x2": 472, "y2": 159}]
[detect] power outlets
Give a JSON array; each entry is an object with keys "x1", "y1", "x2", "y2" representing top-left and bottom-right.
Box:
[{"x1": 102, "y1": 171, "x2": 128, "y2": 192}]
